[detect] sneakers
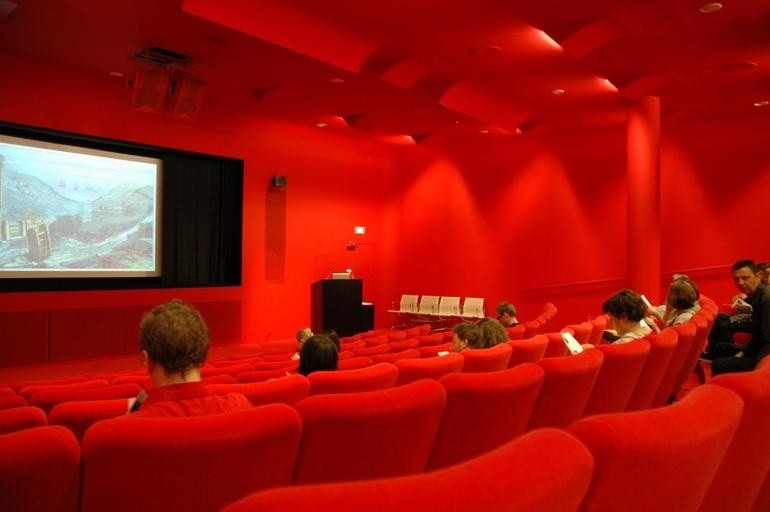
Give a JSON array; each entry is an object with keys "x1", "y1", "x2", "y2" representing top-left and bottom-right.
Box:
[{"x1": 700, "y1": 352, "x2": 713, "y2": 362}]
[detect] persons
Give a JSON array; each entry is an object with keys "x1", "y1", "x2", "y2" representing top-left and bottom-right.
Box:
[
  {"x1": 582, "y1": 260, "x2": 770, "y2": 378},
  {"x1": 139, "y1": 299, "x2": 209, "y2": 386},
  {"x1": 453, "y1": 318, "x2": 510, "y2": 353},
  {"x1": 294, "y1": 328, "x2": 340, "y2": 376},
  {"x1": 496, "y1": 301, "x2": 519, "y2": 327}
]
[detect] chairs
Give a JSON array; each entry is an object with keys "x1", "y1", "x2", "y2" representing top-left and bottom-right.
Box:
[
  {"x1": 20, "y1": 404, "x2": 46, "y2": 429},
  {"x1": 427, "y1": 358, "x2": 547, "y2": 473},
  {"x1": 708, "y1": 353, "x2": 769, "y2": 510},
  {"x1": 75, "y1": 402, "x2": 306, "y2": 512},
  {"x1": 19, "y1": 294, "x2": 727, "y2": 400},
  {"x1": 215, "y1": 423, "x2": 597, "y2": 512},
  {"x1": 48, "y1": 395, "x2": 131, "y2": 427},
  {"x1": 560, "y1": 381, "x2": 748, "y2": 510},
  {"x1": 20, "y1": 425, "x2": 83, "y2": 512},
  {"x1": 285, "y1": 377, "x2": 447, "y2": 487}
]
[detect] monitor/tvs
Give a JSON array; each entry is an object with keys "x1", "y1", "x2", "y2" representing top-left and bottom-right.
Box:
[{"x1": 332, "y1": 273, "x2": 350, "y2": 279}]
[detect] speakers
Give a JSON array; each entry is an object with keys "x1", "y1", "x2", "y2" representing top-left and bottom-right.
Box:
[
  {"x1": 275, "y1": 177, "x2": 286, "y2": 187},
  {"x1": 126, "y1": 63, "x2": 169, "y2": 113},
  {"x1": 164, "y1": 69, "x2": 208, "y2": 123}
]
